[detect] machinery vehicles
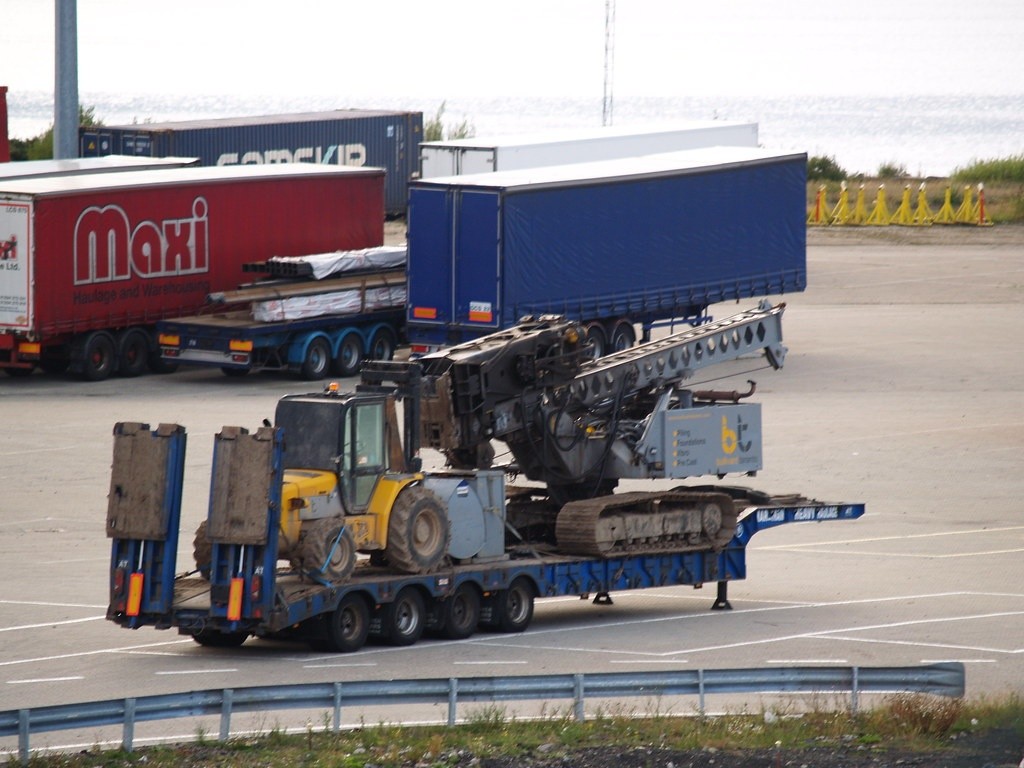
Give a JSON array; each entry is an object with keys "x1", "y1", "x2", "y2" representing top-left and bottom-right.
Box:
[
  {"x1": 403, "y1": 298, "x2": 788, "y2": 561},
  {"x1": 192, "y1": 382, "x2": 511, "y2": 586}
]
